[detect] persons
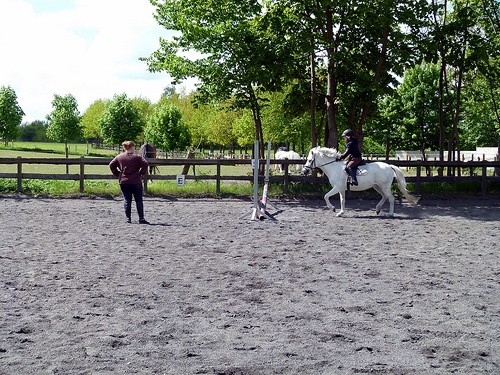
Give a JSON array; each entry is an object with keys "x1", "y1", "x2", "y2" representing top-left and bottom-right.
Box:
[
  {"x1": 109, "y1": 141, "x2": 150, "y2": 224},
  {"x1": 336, "y1": 129, "x2": 362, "y2": 186}
]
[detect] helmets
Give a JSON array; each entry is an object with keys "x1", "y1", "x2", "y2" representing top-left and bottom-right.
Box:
[{"x1": 342, "y1": 129, "x2": 353, "y2": 137}]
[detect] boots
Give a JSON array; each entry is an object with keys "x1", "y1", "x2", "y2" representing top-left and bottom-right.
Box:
[{"x1": 349, "y1": 169, "x2": 358, "y2": 186}]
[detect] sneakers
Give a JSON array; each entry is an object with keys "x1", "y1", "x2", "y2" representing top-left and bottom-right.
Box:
[
  {"x1": 127, "y1": 219, "x2": 131, "y2": 223},
  {"x1": 139, "y1": 219, "x2": 149, "y2": 224}
]
